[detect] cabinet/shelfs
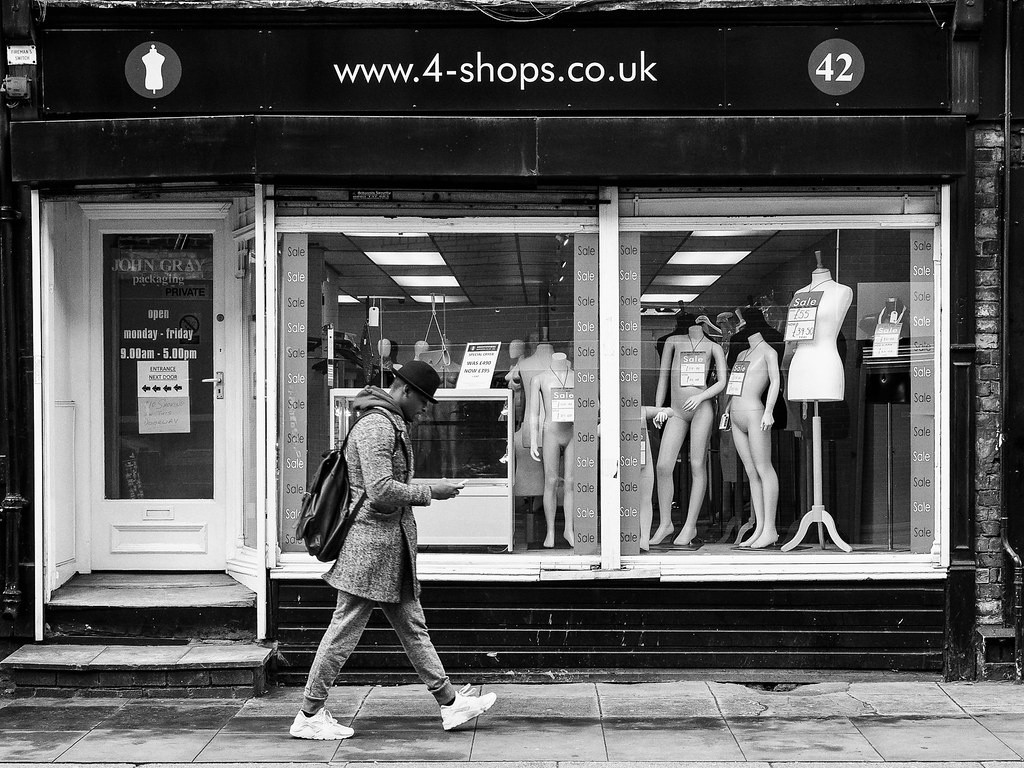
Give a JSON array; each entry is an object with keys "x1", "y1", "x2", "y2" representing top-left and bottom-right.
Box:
[{"x1": 328, "y1": 388, "x2": 514, "y2": 551}]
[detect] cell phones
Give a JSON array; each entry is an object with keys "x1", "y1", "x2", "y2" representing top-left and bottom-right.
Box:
[{"x1": 458, "y1": 478, "x2": 470, "y2": 486}]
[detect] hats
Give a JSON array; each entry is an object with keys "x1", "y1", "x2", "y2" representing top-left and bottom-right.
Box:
[{"x1": 390, "y1": 360, "x2": 440, "y2": 404}]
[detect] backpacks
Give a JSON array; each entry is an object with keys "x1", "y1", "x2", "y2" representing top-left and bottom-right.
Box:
[{"x1": 295, "y1": 409, "x2": 399, "y2": 562}]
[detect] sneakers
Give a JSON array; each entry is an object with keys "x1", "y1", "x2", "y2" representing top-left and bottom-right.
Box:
[
  {"x1": 440, "y1": 683, "x2": 496, "y2": 731},
  {"x1": 289, "y1": 707, "x2": 354, "y2": 741}
]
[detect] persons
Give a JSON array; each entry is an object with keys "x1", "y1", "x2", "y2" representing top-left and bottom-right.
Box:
[
  {"x1": 714, "y1": 309, "x2": 787, "y2": 545},
  {"x1": 515, "y1": 422, "x2": 544, "y2": 497},
  {"x1": 409, "y1": 341, "x2": 433, "y2": 473},
  {"x1": 649, "y1": 325, "x2": 726, "y2": 546},
  {"x1": 801, "y1": 331, "x2": 851, "y2": 440},
  {"x1": 530, "y1": 353, "x2": 574, "y2": 548},
  {"x1": 724, "y1": 332, "x2": 780, "y2": 550},
  {"x1": 520, "y1": 344, "x2": 572, "y2": 447},
  {"x1": 597, "y1": 407, "x2": 674, "y2": 551},
  {"x1": 505, "y1": 339, "x2": 525, "y2": 431},
  {"x1": 436, "y1": 340, "x2": 460, "y2": 478},
  {"x1": 289, "y1": 361, "x2": 498, "y2": 740},
  {"x1": 378, "y1": 339, "x2": 391, "y2": 358},
  {"x1": 780, "y1": 267, "x2": 854, "y2": 402},
  {"x1": 657, "y1": 320, "x2": 716, "y2": 545}
]
